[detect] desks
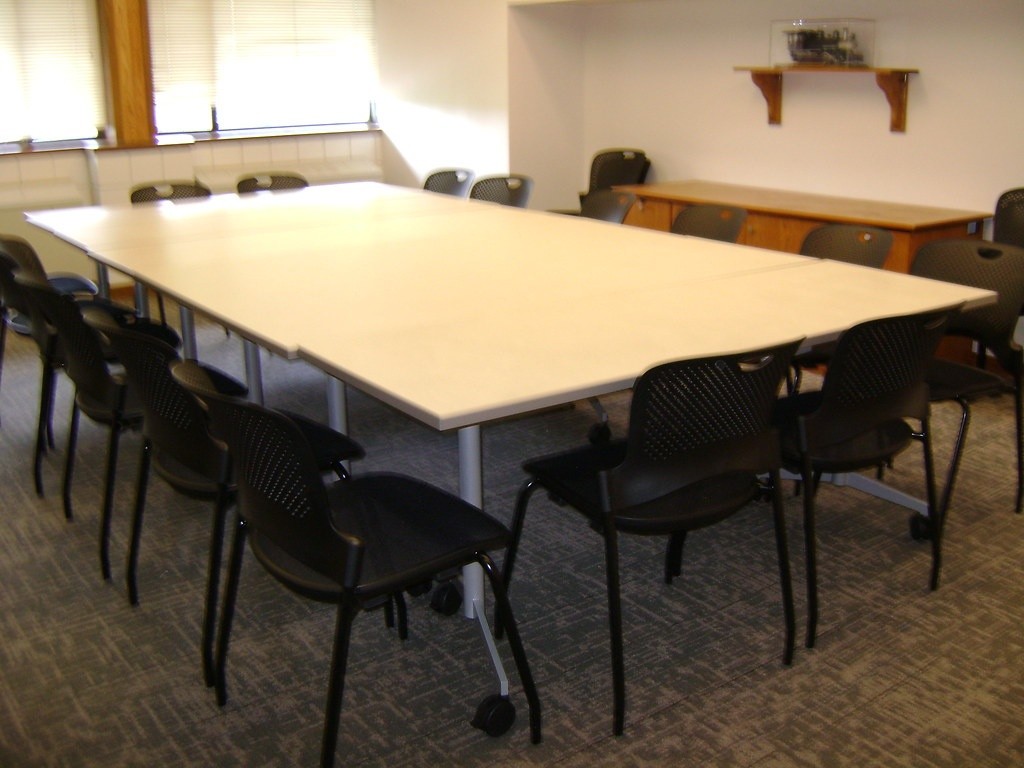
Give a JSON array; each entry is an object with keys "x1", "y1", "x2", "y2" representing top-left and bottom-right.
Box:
[{"x1": 25, "y1": 182, "x2": 998, "y2": 740}]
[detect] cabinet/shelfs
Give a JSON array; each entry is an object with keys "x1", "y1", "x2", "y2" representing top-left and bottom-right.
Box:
[{"x1": 614, "y1": 183, "x2": 995, "y2": 273}]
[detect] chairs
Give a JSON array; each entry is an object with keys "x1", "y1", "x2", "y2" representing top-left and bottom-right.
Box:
[
  {"x1": 492, "y1": 316, "x2": 996, "y2": 735},
  {"x1": 0, "y1": 234, "x2": 541, "y2": 764},
  {"x1": 425, "y1": 148, "x2": 1024, "y2": 505}
]
